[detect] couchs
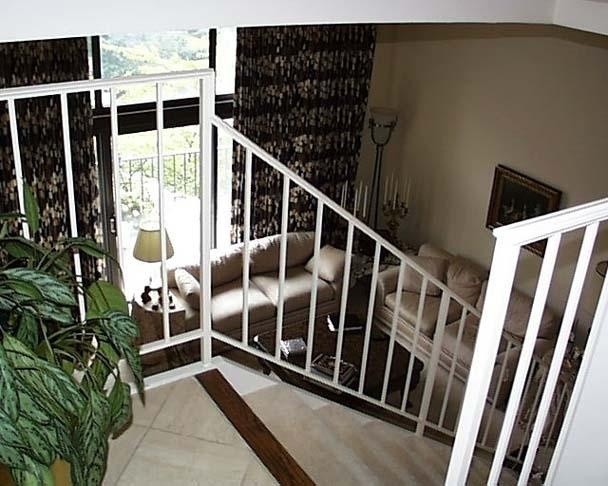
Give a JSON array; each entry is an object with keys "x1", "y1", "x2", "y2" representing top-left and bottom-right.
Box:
[
  {"x1": 373, "y1": 252, "x2": 556, "y2": 408},
  {"x1": 159, "y1": 230, "x2": 358, "y2": 364}
]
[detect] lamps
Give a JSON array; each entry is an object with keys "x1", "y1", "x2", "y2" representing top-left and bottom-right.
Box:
[
  {"x1": 567, "y1": 261, "x2": 608, "y2": 375},
  {"x1": 133, "y1": 219, "x2": 173, "y2": 307},
  {"x1": 362, "y1": 107, "x2": 398, "y2": 250}
]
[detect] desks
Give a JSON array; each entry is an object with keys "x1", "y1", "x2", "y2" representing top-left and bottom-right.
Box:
[{"x1": 517, "y1": 342, "x2": 582, "y2": 448}]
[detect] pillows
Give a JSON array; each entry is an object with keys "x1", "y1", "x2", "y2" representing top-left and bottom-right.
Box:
[
  {"x1": 403, "y1": 240, "x2": 555, "y2": 351},
  {"x1": 161, "y1": 229, "x2": 346, "y2": 310}
]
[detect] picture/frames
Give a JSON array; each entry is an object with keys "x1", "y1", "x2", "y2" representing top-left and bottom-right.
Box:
[{"x1": 484, "y1": 164, "x2": 562, "y2": 258}]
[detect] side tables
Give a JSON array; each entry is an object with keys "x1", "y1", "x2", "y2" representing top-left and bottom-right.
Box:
[
  {"x1": 334, "y1": 229, "x2": 393, "y2": 286},
  {"x1": 131, "y1": 284, "x2": 186, "y2": 365}
]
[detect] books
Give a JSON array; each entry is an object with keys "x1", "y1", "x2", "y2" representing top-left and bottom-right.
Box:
[{"x1": 280, "y1": 310, "x2": 362, "y2": 395}]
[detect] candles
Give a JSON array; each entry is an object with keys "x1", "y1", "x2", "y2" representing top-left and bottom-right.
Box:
[{"x1": 340, "y1": 171, "x2": 412, "y2": 221}]
